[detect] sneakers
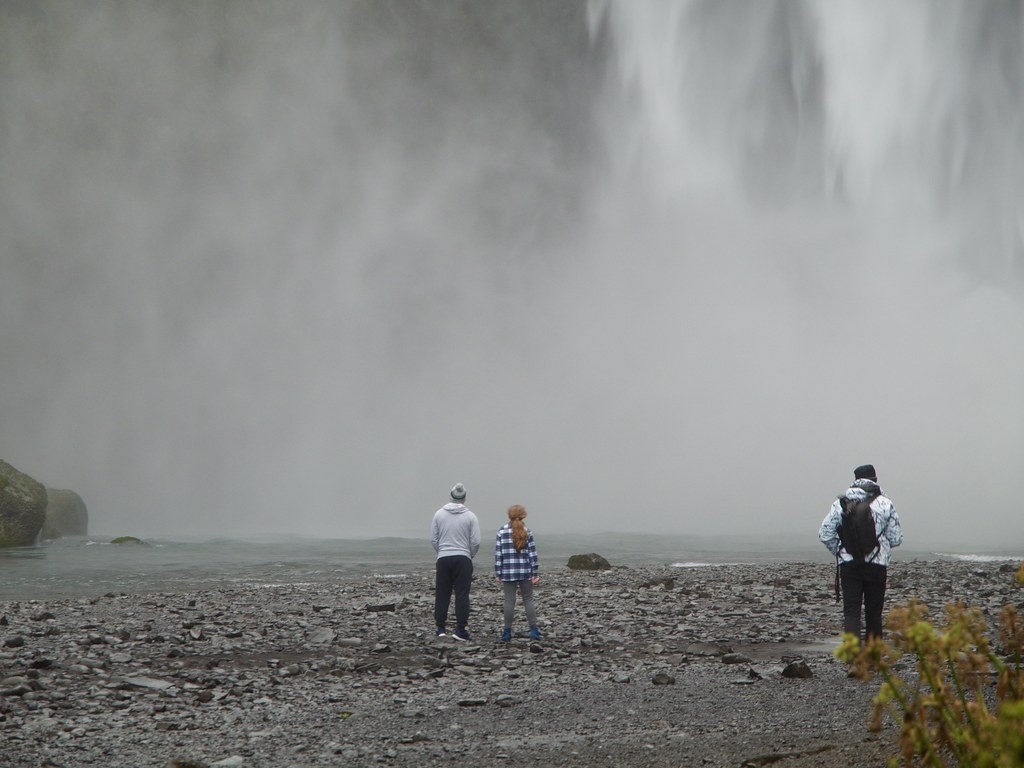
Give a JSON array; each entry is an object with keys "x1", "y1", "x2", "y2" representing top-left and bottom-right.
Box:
[
  {"x1": 451, "y1": 627, "x2": 473, "y2": 641},
  {"x1": 436, "y1": 626, "x2": 446, "y2": 637}
]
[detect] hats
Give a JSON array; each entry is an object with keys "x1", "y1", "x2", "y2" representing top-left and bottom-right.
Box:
[
  {"x1": 854, "y1": 465, "x2": 877, "y2": 482},
  {"x1": 449, "y1": 483, "x2": 467, "y2": 503}
]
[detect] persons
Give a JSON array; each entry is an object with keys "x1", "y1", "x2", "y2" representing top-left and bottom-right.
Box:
[
  {"x1": 819, "y1": 465, "x2": 904, "y2": 660},
  {"x1": 494, "y1": 504, "x2": 543, "y2": 643},
  {"x1": 431, "y1": 483, "x2": 481, "y2": 641}
]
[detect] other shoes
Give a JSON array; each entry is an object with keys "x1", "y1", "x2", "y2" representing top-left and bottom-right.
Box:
[
  {"x1": 846, "y1": 663, "x2": 862, "y2": 677},
  {"x1": 502, "y1": 628, "x2": 511, "y2": 640},
  {"x1": 530, "y1": 626, "x2": 539, "y2": 639}
]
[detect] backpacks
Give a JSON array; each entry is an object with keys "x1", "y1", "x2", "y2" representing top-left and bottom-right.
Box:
[{"x1": 836, "y1": 492, "x2": 888, "y2": 565}]
